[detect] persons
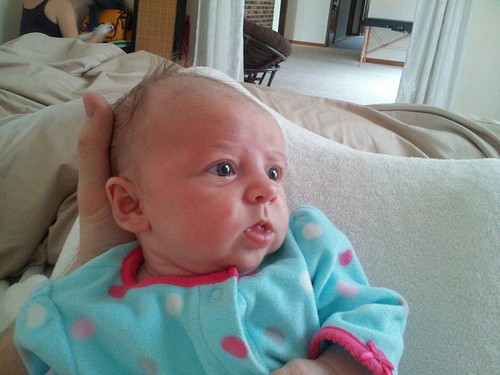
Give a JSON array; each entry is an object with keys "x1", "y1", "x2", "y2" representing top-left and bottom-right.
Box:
[
  {"x1": 19, "y1": 0, "x2": 105, "y2": 45},
  {"x1": 0, "y1": 72, "x2": 409, "y2": 375}
]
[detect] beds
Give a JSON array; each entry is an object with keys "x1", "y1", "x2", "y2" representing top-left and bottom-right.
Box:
[{"x1": 0, "y1": 34, "x2": 500, "y2": 332}]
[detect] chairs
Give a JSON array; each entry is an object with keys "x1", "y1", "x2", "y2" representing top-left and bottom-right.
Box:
[{"x1": 242, "y1": 17, "x2": 292, "y2": 87}]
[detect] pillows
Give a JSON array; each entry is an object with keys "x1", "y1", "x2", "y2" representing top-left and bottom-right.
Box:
[{"x1": 48, "y1": 68, "x2": 499, "y2": 374}]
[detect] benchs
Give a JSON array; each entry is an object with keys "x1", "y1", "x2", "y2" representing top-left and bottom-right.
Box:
[{"x1": 359, "y1": 17, "x2": 413, "y2": 66}]
